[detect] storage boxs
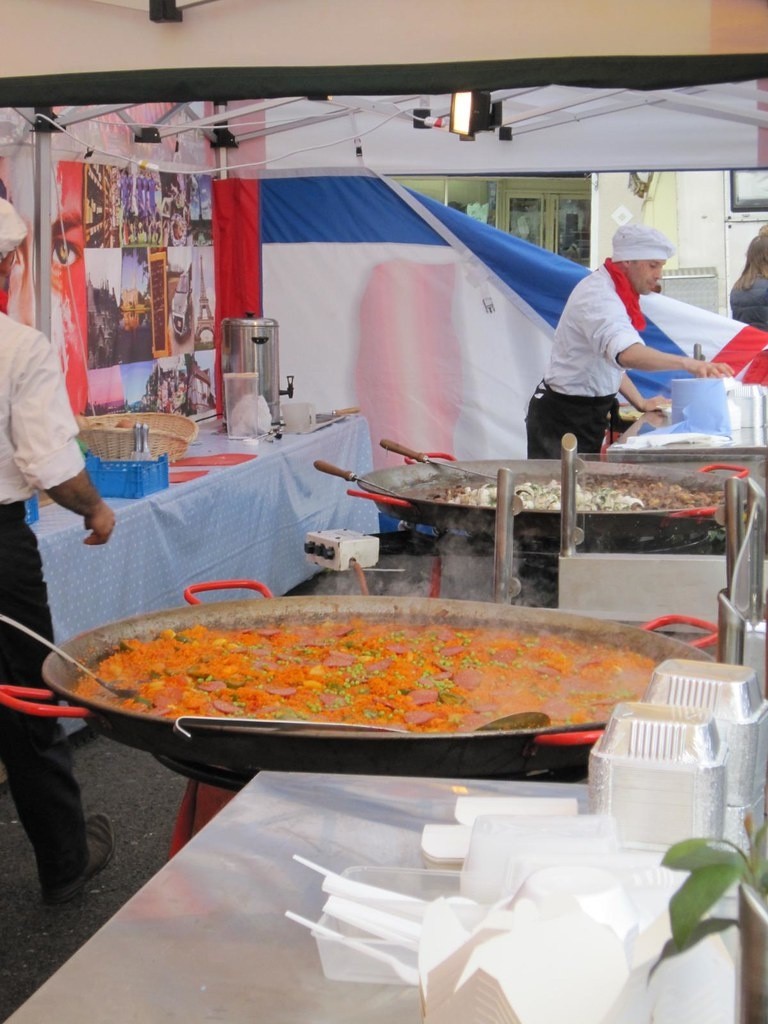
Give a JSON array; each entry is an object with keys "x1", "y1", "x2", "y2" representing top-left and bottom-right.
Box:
[{"x1": 84, "y1": 449, "x2": 169, "y2": 499}]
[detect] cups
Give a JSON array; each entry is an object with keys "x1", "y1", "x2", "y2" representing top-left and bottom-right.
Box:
[
  {"x1": 281, "y1": 401, "x2": 317, "y2": 433},
  {"x1": 224, "y1": 372, "x2": 258, "y2": 439}
]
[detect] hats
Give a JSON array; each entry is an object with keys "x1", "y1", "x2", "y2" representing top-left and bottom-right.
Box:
[
  {"x1": 0, "y1": 198, "x2": 27, "y2": 271},
  {"x1": 611, "y1": 223, "x2": 677, "y2": 263}
]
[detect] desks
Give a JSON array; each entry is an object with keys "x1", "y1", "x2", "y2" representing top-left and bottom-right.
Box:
[
  {"x1": 24, "y1": 409, "x2": 381, "y2": 740},
  {"x1": 2, "y1": 767, "x2": 747, "y2": 1024}
]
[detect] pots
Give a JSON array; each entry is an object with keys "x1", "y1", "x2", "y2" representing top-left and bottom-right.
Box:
[
  {"x1": 346, "y1": 452, "x2": 749, "y2": 550},
  {"x1": 42, "y1": 583, "x2": 716, "y2": 789}
]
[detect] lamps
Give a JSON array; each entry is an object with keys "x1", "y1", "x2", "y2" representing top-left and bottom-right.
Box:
[{"x1": 449, "y1": 91, "x2": 496, "y2": 136}]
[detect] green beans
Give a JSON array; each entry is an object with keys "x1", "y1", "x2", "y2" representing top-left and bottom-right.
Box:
[{"x1": 195, "y1": 630, "x2": 632, "y2": 725}]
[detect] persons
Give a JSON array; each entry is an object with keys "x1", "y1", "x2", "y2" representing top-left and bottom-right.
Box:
[
  {"x1": 731, "y1": 225, "x2": 768, "y2": 334},
  {"x1": 517, "y1": 220, "x2": 735, "y2": 590},
  {"x1": 1, "y1": 198, "x2": 118, "y2": 909}
]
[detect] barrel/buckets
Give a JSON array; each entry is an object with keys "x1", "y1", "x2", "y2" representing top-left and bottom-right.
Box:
[{"x1": 222, "y1": 318, "x2": 294, "y2": 432}]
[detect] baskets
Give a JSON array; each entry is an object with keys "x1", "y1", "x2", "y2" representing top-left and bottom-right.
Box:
[
  {"x1": 83, "y1": 448, "x2": 170, "y2": 500},
  {"x1": 74, "y1": 412, "x2": 200, "y2": 463},
  {"x1": 23, "y1": 491, "x2": 39, "y2": 526}
]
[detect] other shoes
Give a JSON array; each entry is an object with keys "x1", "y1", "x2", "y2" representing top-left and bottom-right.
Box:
[
  {"x1": 517, "y1": 554, "x2": 555, "y2": 583},
  {"x1": 41, "y1": 812, "x2": 116, "y2": 905},
  {"x1": 538, "y1": 556, "x2": 559, "y2": 590}
]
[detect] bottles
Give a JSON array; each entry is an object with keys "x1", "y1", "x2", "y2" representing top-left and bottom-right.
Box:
[{"x1": 130, "y1": 422, "x2": 151, "y2": 462}]
